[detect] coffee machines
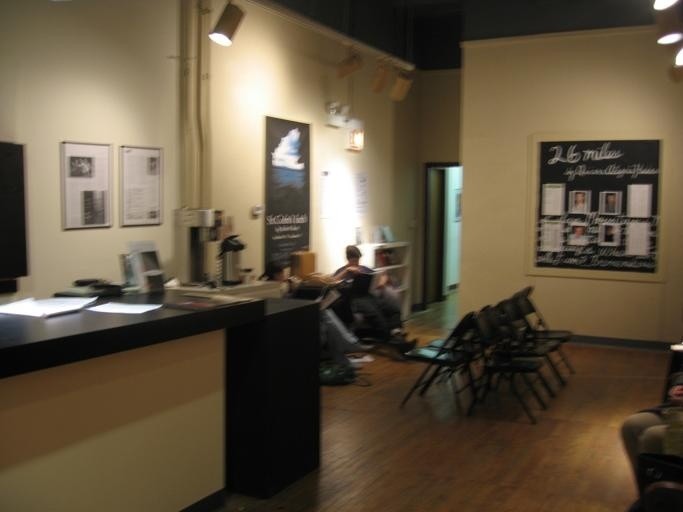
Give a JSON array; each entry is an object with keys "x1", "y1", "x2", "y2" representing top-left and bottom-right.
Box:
[{"x1": 171, "y1": 207, "x2": 246, "y2": 287}]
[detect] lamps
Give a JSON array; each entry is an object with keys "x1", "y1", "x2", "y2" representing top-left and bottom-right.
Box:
[{"x1": 206, "y1": 4, "x2": 245, "y2": 46}]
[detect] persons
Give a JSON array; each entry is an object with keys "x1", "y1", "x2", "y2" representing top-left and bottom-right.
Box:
[
  {"x1": 568, "y1": 192, "x2": 618, "y2": 242},
  {"x1": 266, "y1": 245, "x2": 417, "y2": 355},
  {"x1": 620, "y1": 371, "x2": 683, "y2": 472}
]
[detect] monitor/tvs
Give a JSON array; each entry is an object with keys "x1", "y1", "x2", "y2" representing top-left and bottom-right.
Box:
[{"x1": 0, "y1": 141, "x2": 26, "y2": 297}]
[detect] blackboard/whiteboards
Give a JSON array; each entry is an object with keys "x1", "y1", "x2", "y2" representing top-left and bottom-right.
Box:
[{"x1": 524, "y1": 134, "x2": 665, "y2": 285}]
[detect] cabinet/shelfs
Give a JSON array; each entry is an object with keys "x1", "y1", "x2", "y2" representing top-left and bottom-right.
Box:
[{"x1": 355, "y1": 242, "x2": 411, "y2": 322}]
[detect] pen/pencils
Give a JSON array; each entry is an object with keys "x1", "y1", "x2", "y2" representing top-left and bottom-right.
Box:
[{"x1": 181, "y1": 295, "x2": 209, "y2": 299}]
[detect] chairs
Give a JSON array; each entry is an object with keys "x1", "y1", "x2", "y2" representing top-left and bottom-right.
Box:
[{"x1": 400, "y1": 284, "x2": 574, "y2": 427}]
